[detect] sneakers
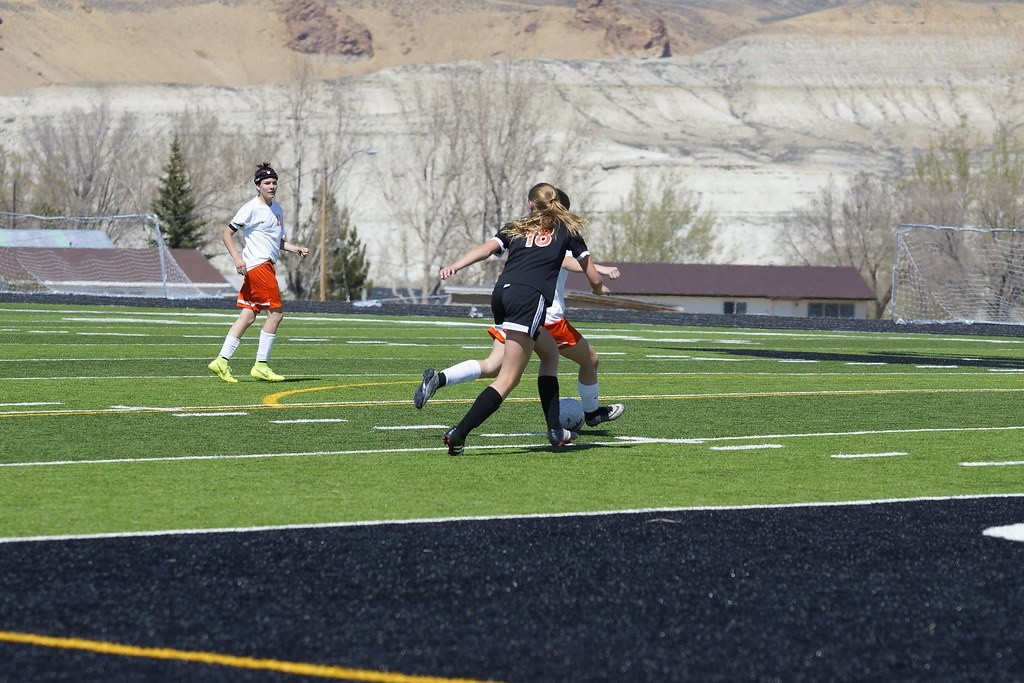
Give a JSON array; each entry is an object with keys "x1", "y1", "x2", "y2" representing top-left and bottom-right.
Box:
[
  {"x1": 250, "y1": 360, "x2": 286, "y2": 382},
  {"x1": 443, "y1": 424, "x2": 464, "y2": 456},
  {"x1": 584, "y1": 403, "x2": 625, "y2": 427},
  {"x1": 208, "y1": 355, "x2": 239, "y2": 382},
  {"x1": 546, "y1": 428, "x2": 580, "y2": 448},
  {"x1": 414, "y1": 367, "x2": 439, "y2": 409}
]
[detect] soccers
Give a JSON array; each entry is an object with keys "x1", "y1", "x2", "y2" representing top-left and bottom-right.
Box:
[{"x1": 559, "y1": 398, "x2": 584, "y2": 432}]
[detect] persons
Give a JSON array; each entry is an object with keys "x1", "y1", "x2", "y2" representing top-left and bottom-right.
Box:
[
  {"x1": 412, "y1": 182, "x2": 626, "y2": 457},
  {"x1": 207, "y1": 162, "x2": 310, "y2": 382}
]
